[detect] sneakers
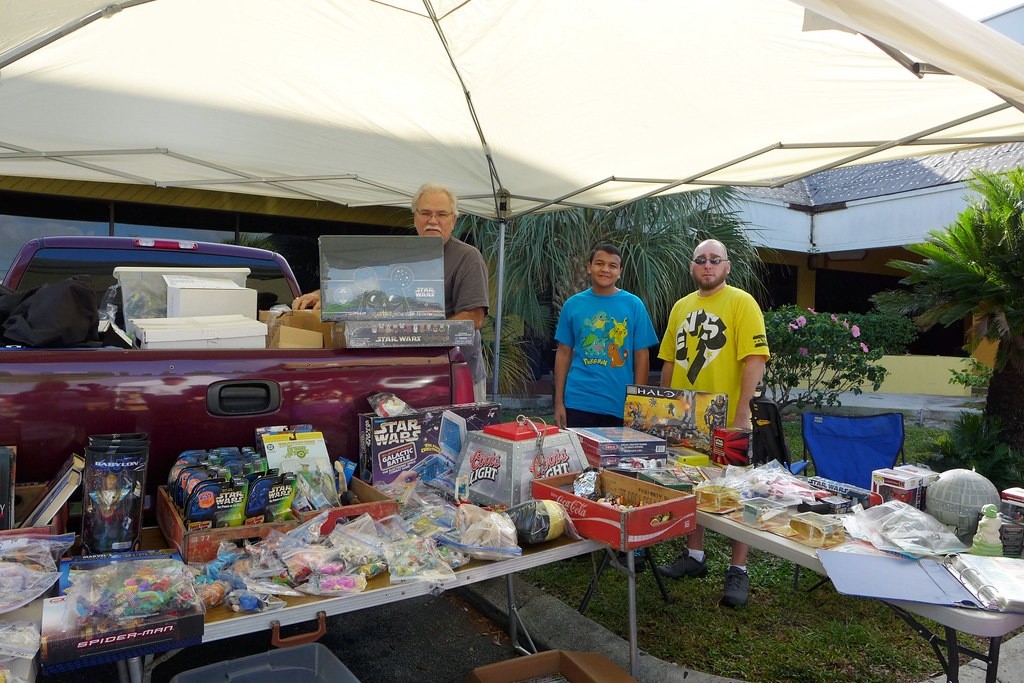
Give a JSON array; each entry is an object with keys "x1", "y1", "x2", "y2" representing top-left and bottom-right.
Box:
[
  {"x1": 721, "y1": 566, "x2": 749, "y2": 608},
  {"x1": 657, "y1": 547, "x2": 708, "y2": 581}
]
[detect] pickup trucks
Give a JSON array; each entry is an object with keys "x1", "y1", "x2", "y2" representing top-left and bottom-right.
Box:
[{"x1": 0, "y1": 236, "x2": 485, "y2": 523}]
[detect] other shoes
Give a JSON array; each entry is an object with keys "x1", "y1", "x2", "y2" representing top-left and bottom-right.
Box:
[{"x1": 610, "y1": 551, "x2": 646, "y2": 573}]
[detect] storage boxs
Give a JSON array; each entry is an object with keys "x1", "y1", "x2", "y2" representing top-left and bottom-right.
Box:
[
  {"x1": 867, "y1": 465, "x2": 942, "y2": 515},
  {"x1": 1, "y1": 234, "x2": 748, "y2": 683},
  {"x1": 1002, "y1": 486, "x2": 1024, "y2": 523}
]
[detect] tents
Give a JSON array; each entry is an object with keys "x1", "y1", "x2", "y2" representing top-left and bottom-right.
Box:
[{"x1": 0, "y1": 0, "x2": 1024, "y2": 406}]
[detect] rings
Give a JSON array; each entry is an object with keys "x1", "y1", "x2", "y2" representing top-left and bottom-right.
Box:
[{"x1": 296, "y1": 297, "x2": 299, "y2": 299}]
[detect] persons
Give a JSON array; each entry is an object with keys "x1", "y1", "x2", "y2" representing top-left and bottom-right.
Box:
[
  {"x1": 554, "y1": 245, "x2": 660, "y2": 566},
  {"x1": 657, "y1": 239, "x2": 770, "y2": 606},
  {"x1": 291, "y1": 178, "x2": 490, "y2": 401}
]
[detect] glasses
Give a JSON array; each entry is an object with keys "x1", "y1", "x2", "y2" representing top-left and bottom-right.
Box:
[
  {"x1": 416, "y1": 208, "x2": 453, "y2": 219},
  {"x1": 693, "y1": 258, "x2": 726, "y2": 265}
]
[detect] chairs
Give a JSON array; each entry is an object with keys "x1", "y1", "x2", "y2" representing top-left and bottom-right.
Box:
[{"x1": 788, "y1": 411, "x2": 912, "y2": 595}]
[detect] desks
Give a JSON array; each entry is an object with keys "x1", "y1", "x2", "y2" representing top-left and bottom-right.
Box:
[
  {"x1": 0, "y1": 563, "x2": 58, "y2": 682},
  {"x1": 113, "y1": 546, "x2": 638, "y2": 682},
  {"x1": 696, "y1": 508, "x2": 1024, "y2": 682}
]
